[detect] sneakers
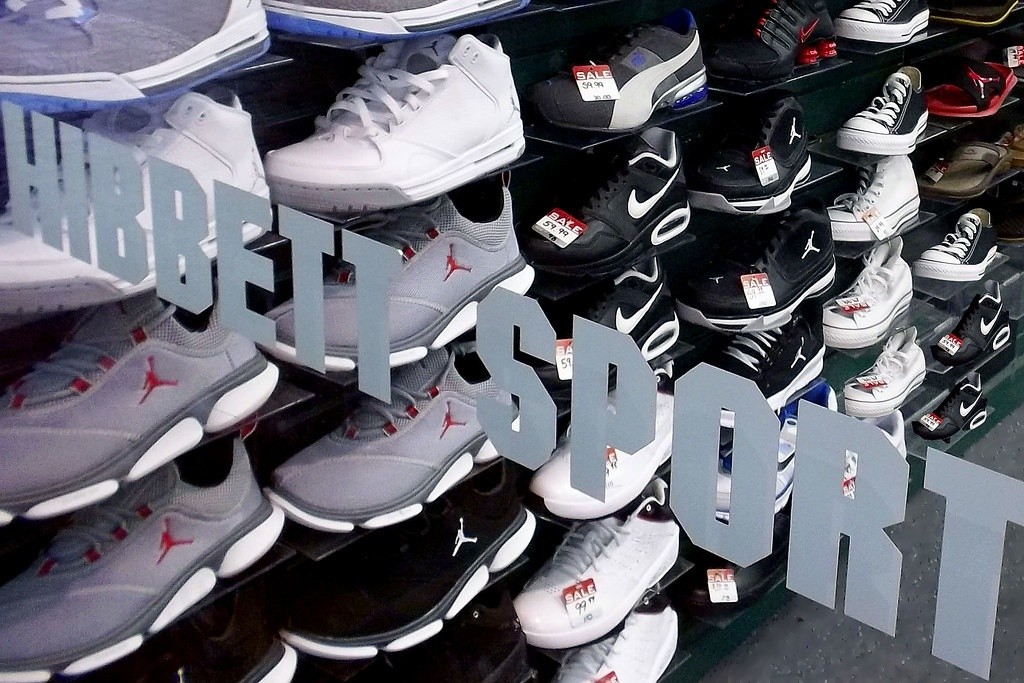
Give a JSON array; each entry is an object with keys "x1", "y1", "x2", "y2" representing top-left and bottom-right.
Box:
[
  {"x1": 50, "y1": 588, "x2": 298, "y2": 683},
  {"x1": 0, "y1": 1, "x2": 272, "y2": 114},
  {"x1": 0, "y1": 279, "x2": 279, "y2": 527},
  {"x1": 1, "y1": 85, "x2": 272, "y2": 320},
  {"x1": 264, "y1": 1, "x2": 1023, "y2": 682},
  {"x1": 1, "y1": 433, "x2": 288, "y2": 683}
]
